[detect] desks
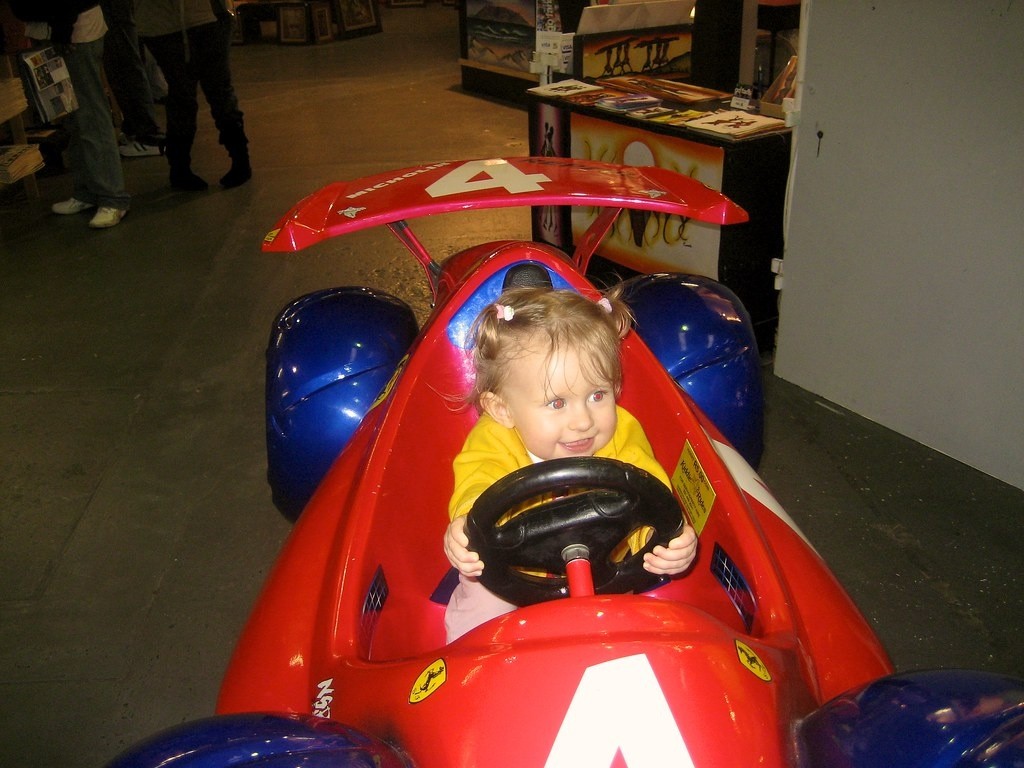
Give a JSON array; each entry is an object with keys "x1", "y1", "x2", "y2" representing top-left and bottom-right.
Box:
[{"x1": 527, "y1": 78, "x2": 793, "y2": 344}]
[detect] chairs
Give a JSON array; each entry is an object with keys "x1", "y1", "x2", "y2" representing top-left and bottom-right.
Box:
[{"x1": 347, "y1": 245, "x2": 788, "y2": 654}]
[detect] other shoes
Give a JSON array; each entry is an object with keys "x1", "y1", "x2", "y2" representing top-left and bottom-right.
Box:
[
  {"x1": 170, "y1": 168, "x2": 208, "y2": 192},
  {"x1": 220, "y1": 165, "x2": 252, "y2": 188}
]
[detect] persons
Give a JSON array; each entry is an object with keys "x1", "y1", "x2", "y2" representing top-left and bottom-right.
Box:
[
  {"x1": 444, "y1": 283, "x2": 698, "y2": 643},
  {"x1": 1, "y1": 0, "x2": 253, "y2": 229}
]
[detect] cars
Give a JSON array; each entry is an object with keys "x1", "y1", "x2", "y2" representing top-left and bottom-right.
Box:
[{"x1": 96, "y1": 156, "x2": 1024, "y2": 768}]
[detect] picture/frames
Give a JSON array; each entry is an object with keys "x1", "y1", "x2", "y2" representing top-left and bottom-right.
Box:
[{"x1": 230, "y1": 0, "x2": 427, "y2": 46}]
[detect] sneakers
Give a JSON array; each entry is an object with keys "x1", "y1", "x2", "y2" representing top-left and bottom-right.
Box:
[
  {"x1": 119, "y1": 141, "x2": 166, "y2": 156},
  {"x1": 119, "y1": 132, "x2": 135, "y2": 144},
  {"x1": 51, "y1": 196, "x2": 95, "y2": 214},
  {"x1": 89, "y1": 206, "x2": 126, "y2": 228}
]
